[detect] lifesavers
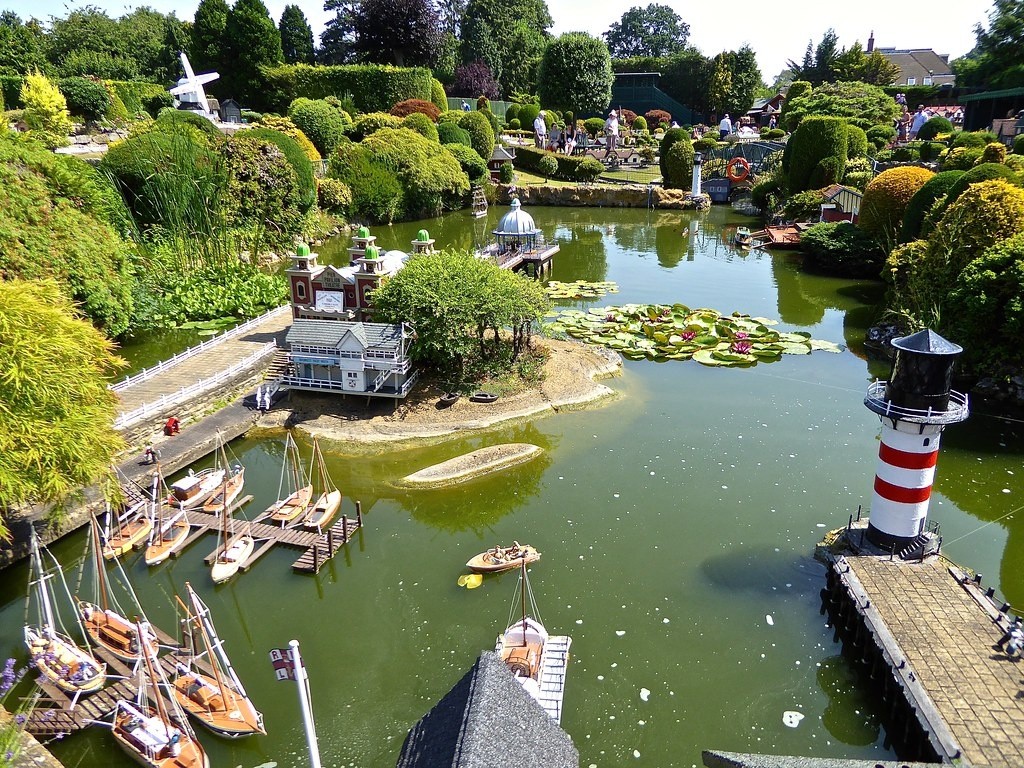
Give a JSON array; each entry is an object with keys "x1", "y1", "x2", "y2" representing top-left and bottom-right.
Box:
[
  {"x1": 727, "y1": 157, "x2": 749, "y2": 182},
  {"x1": 349, "y1": 380, "x2": 356, "y2": 387}
]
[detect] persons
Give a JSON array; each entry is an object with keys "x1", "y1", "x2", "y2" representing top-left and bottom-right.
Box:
[
  {"x1": 461, "y1": 99, "x2": 471, "y2": 111},
  {"x1": 769, "y1": 115, "x2": 776, "y2": 130},
  {"x1": 891, "y1": 93, "x2": 955, "y2": 145},
  {"x1": 603, "y1": 109, "x2": 619, "y2": 151},
  {"x1": 492, "y1": 541, "x2": 529, "y2": 561},
  {"x1": 672, "y1": 121, "x2": 679, "y2": 128},
  {"x1": 715, "y1": 112, "x2": 746, "y2": 143},
  {"x1": 533, "y1": 108, "x2": 577, "y2": 158}
]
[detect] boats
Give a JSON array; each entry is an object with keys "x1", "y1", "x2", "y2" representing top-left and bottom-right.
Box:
[
  {"x1": 441, "y1": 391, "x2": 460, "y2": 405},
  {"x1": 465, "y1": 544, "x2": 541, "y2": 572},
  {"x1": 167, "y1": 468, "x2": 227, "y2": 511},
  {"x1": 472, "y1": 392, "x2": 499, "y2": 402},
  {"x1": 733, "y1": 226, "x2": 751, "y2": 245}
]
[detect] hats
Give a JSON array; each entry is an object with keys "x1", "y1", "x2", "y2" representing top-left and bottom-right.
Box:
[
  {"x1": 146, "y1": 446, "x2": 151, "y2": 449},
  {"x1": 609, "y1": 109, "x2": 618, "y2": 117}
]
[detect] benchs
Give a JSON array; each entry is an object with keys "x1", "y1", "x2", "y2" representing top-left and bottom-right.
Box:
[{"x1": 556, "y1": 144, "x2": 602, "y2": 157}]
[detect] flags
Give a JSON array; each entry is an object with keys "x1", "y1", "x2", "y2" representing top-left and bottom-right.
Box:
[{"x1": 269, "y1": 648, "x2": 309, "y2": 681}]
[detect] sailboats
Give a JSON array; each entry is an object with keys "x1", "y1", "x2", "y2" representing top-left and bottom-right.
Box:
[
  {"x1": 202, "y1": 426, "x2": 245, "y2": 513},
  {"x1": 270, "y1": 431, "x2": 313, "y2": 523},
  {"x1": 302, "y1": 435, "x2": 342, "y2": 532},
  {"x1": 73, "y1": 507, "x2": 160, "y2": 662},
  {"x1": 23, "y1": 524, "x2": 108, "y2": 696},
  {"x1": 102, "y1": 464, "x2": 152, "y2": 562},
  {"x1": 169, "y1": 581, "x2": 268, "y2": 738},
  {"x1": 111, "y1": 616, "x2": 212, "y2": 768},
  {"x1": 494, "y1": 559, "x2": 549, "y2": 691},
  {"x1": 212, "y1": 476, "x2": 255, "y2": 583},
  {"x1": 145, "y1": 472, "x2": 191, "y2": 567}
]
